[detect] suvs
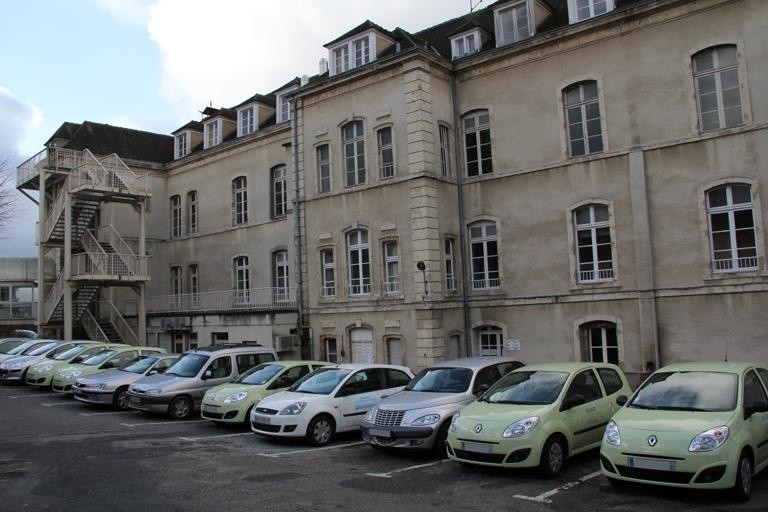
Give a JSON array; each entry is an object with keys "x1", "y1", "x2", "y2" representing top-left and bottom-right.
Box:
[{"x1": 126, "y1": 346, "x2": 280, "y2": 421}]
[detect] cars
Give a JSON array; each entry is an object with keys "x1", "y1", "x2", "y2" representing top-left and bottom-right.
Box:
[
  {"x1": 51, "y1": 345, "x2": 167, "y2": 398},
  {"x1": 248, "y1": 361, "x2": 421, "y2": 447},
  {"x1": 200, "y1": 360, "x2": 337, "y2": 432},
  {"x1": 445, "y1": 361, "x2": 635, "y2": 477},
  {"x1": 360, "y1": 356, "x2": 530, "y2": 461},
  {"x1": 598, "y1": 341, "x2": 767, "y2": 503},
  {"x1": 0, "y1": 338, "x2": 42, "y2": 365},
  {"x1": 71, "y1": 352, "x2": 182, "y2": 412},
  {"x1": 0, "y1": 341, "x2": 69, "y2": 386},
  {"x1": 24, "y1": 343, "x2": 132, "y2": 391}
]
